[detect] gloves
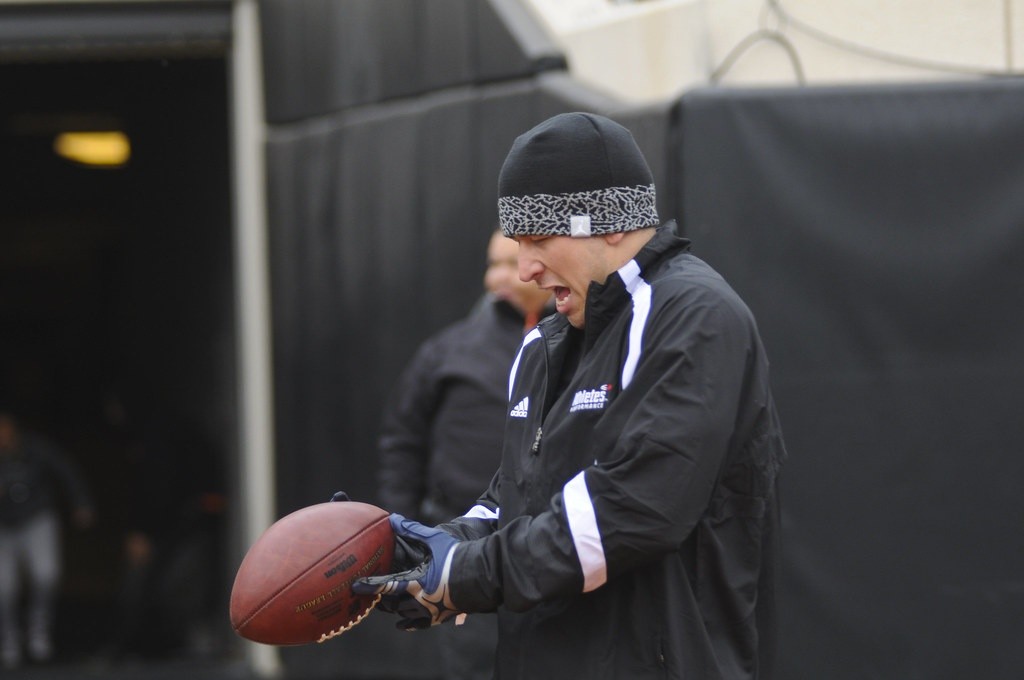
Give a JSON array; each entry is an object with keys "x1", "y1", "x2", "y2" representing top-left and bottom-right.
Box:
[{"x1": 330, "y1": 492, "x2": 501, "y2": 631}]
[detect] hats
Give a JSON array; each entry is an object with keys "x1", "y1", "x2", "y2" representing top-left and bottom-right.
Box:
[{"x1": 497, "y1": 111, "x2": 662, "y2": 237}]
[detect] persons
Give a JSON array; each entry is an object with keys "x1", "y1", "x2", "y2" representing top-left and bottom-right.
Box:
[
  {"x1": 0, "y1": 405, "x2": 96, "y2": 680},
  {"x1": 377, "y1": 226, "x2": 558, "y2": 680},
  {"x1": 329, "y1": 112, "x2": 788, "y2": 680}
]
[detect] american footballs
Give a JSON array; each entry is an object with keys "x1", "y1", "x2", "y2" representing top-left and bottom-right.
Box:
[{"x1": 227, "y1": 499, "x2": 396, "y2": 647}]
